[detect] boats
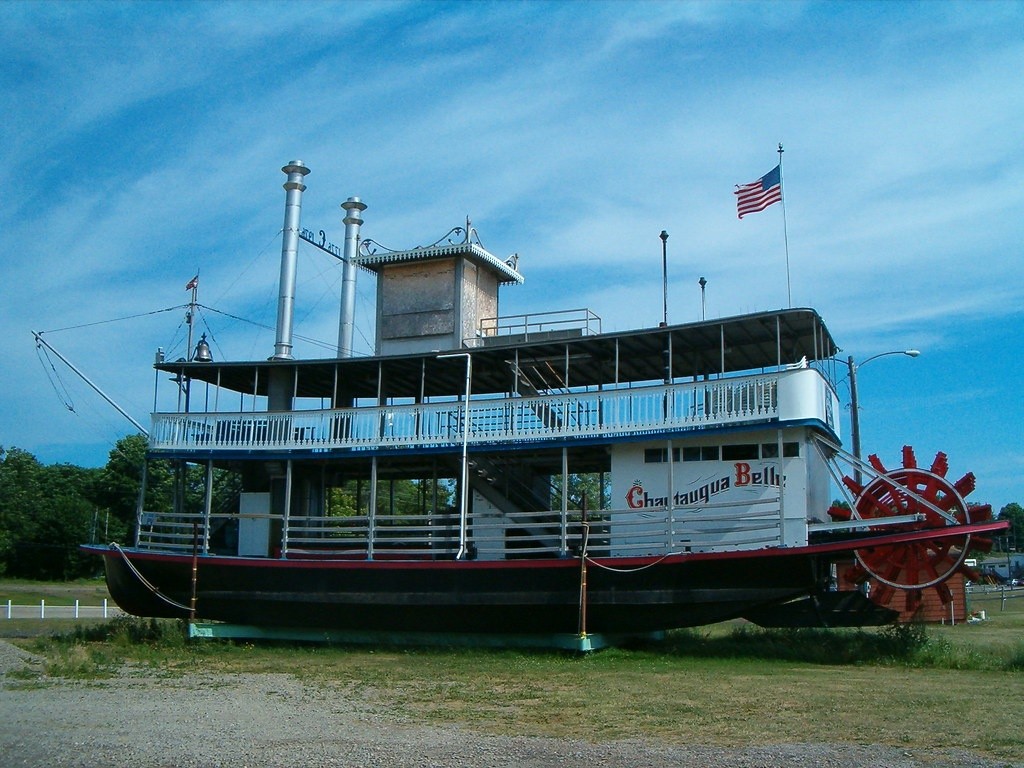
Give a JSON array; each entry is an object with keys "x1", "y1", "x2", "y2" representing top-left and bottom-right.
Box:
[{"x1": 32, "y1": 157, "x2": 1009, "y2": 645}]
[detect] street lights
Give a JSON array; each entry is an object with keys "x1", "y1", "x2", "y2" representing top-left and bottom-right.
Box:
[{"x1": 849, "y1": 350, "x2": 921, "y2": 495}]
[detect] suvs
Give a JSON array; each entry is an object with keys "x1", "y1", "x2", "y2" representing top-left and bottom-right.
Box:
[{"x1": 1007, "y1": 579, "x2": 1018, "y2": 586}]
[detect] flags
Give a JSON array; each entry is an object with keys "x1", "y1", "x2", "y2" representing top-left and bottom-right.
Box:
[
  {"x1": 186, "y1": 275, "x2": 198, "y2": 292},
  {"x1": 733, "y1": 164, "x2": 785, "y2": 219}
]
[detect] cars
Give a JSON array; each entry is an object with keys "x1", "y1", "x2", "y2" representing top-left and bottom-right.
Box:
[{"x1": 965, "y1": 580, "x2": 973, "y2": 586}]
